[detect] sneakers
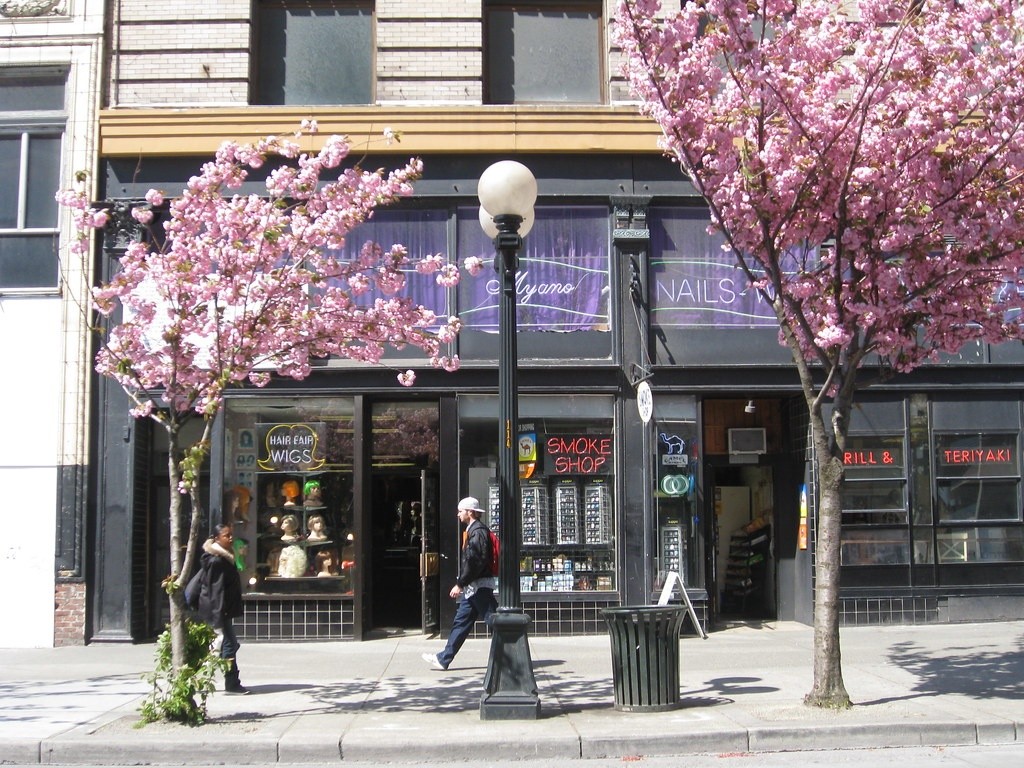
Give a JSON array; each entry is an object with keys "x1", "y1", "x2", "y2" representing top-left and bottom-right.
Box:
[{"x1": 421, "y1": 654, "x2": 444, "y2": 669}]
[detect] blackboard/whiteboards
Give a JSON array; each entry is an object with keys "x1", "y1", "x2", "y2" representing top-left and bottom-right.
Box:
[{"x1": 658, "y1": 571, "x2": 706, "y2": 639}]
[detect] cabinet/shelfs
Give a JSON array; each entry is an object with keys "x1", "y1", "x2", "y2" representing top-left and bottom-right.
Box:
[
  {"x1": 660, "y1": 518, "x2": 689, "y2": 586},
  {"x1": 257, "y1": 472, "x2": 347, "y2": 581},
  {"x1": 489, "y1": 480, "x2": 619, "y2": 592},
  {"x1": 724, "y1": 524, "x2": 771, "y2": 615}
]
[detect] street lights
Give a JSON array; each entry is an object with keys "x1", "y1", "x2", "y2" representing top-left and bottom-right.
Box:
[{"x1": 477, "y1": 159, "x2": 542, "y2": 720}]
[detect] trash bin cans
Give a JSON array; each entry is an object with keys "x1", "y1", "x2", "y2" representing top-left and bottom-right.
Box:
[{"x1": 600, "y1": 605, "x2": 689, "y2": 713}]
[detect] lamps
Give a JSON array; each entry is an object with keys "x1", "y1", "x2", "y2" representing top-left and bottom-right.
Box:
[{"x1": 745, "y1": 399, "x2": 756, "y2": 413}]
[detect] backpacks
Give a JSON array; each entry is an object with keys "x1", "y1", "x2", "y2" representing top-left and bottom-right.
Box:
[{"x1": 468, "y1": 525, "x2": 500, "y2": 576}]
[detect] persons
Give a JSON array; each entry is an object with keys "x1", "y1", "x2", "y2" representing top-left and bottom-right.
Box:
[
  {"x1": 281, "y1": 480, "x2": 324, "y2": 507},
  {"x1": 421, "y1": 497, "x2": 498, "y2": 670},
  {"x1": 278, "y1": 545, "x2": 339, "y2": 577},
  {"x1": 281, "y1": 514, "x2": 327, "y2": 542},
  {"x1": 223, "y1": 485, "x2": 251, "y2": 571},
  {"x1": 199, "y1": 524, "x2": 250, "y2": 694}
]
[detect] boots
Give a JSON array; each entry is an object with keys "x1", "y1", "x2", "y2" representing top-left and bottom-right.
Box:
[{"x1": 224, "y1": 670, "x2": 250, "y2": 695}]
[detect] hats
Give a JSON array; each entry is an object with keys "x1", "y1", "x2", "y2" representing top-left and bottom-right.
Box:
[{"x1": 458, "y1": 497, "x2": 486, "y2": 513}]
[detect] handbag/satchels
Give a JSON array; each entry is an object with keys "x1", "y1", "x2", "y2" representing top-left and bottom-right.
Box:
[{"x1": 184, "y1": 568, "x2": 206, "y2": 611}]
